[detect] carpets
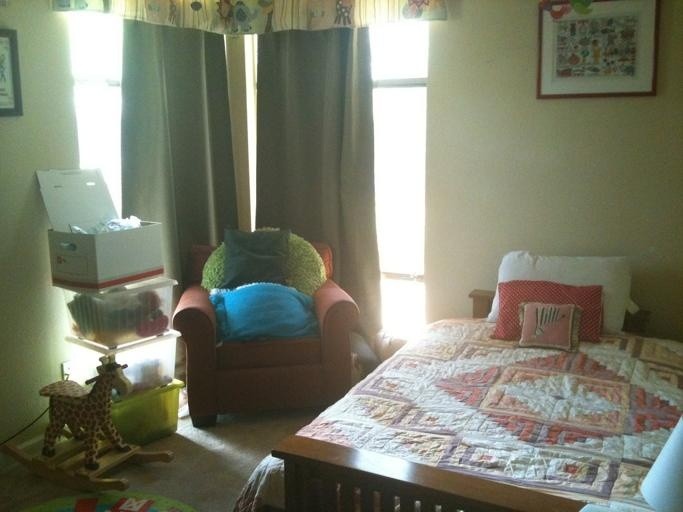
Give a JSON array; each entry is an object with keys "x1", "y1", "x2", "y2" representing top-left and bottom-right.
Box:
[{"x1": 23, "y1": 490, "x2": 197, "y2": 512}]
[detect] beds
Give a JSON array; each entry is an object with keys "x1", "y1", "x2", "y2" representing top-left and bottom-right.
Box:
[{"x1": 231, "y1": 289, "x2": 683, "y2": 512}]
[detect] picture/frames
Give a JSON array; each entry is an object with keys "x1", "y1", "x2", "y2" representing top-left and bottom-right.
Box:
[
  {"x1": 535, "y1": 0, "x2": 659, "y2": 98},
  {"x1": 0, "y1": 28, "x2": 23, "y2": 117}
]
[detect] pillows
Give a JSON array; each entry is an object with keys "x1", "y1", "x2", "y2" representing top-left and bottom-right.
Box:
[
  {"x1": 199, "y1": 228, "x2": 328, "y2": 343},
  {"x1": 486, "y1": 250, "x2": 632, "y2": 352}
]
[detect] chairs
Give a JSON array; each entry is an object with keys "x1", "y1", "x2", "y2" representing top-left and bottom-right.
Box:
[{"x1": 172, "y1": 240, "x2": 360, "y2": 428}]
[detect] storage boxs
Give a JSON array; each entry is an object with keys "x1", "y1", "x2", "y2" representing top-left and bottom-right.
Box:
[{"x1": 47, "y1": 219, "x2": 187, "y2": 447}]
